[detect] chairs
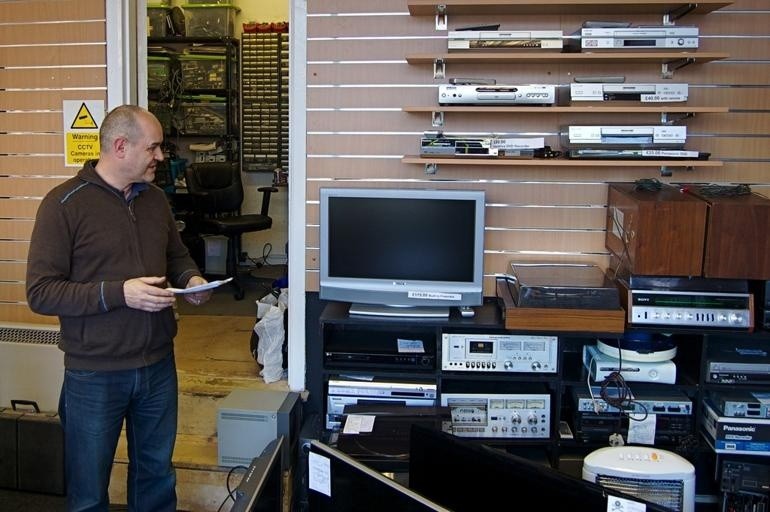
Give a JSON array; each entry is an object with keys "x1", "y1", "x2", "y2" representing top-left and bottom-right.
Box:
[{"x1": 191, "y1": 163, "x2": 277, "y2": 300}]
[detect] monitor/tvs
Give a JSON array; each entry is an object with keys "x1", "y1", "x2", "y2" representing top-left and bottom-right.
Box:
[{"x1": 317, "y1": 186, "x2": 485, "y2": 319}]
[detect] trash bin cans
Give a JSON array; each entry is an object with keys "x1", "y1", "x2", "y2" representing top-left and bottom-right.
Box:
[{"x1": 251, "y1": 289, "x2": 287, "y2": 369}]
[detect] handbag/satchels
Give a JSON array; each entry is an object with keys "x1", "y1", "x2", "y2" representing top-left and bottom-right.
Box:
[{"x1": 250, "y1": 288, "x2": 287, "y2": 384}]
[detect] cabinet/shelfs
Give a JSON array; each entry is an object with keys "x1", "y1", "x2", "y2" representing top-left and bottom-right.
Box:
[
  {"x1": 402, "y1": 0, "x2": 728, "y2": 179},
  {"x1": 147, "y1": 37, "x2": 240, "y2": 160},
  {"x1": 319, "y1": 301, "x2": 770, "y2": 511}
]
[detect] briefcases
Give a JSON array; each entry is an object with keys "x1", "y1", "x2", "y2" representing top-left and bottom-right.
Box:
[{"x1": 0, "y1": 400, "x2": 65, "y2": 496}]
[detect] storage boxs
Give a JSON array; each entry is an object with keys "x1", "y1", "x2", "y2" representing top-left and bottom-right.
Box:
[
  {"x1": 179, "y1": 96, "x2": 225, "y2": 134},
  {"x1": 183, "y1": 4, "x2": 240, "y2": 37},
  {"x1": 607, "y1": 181, "x2": 706, "y2": 277},
  {"x1": 148, "y1": 95, "x2": 173, "y2": 134},
  {"x1": 148, "y1": 56, "x2": 170, "y2": 90},
  {"x1": 179, "y1": 56, "x2": 225, "y2": 89},
  {"x1": 147, "y1": 4, "x2": 171, "y2": 37},
  {"x1": 679, "y1": 184, "x2": 769, "y2": 279}
]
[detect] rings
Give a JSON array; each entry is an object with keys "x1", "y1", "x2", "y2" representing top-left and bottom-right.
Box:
[{"x1": 197, "y1": 300, "x2": 201, "y2": 304}]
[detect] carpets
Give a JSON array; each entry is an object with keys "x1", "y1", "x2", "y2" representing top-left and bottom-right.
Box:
[{"x1": 176, "y1": 265, "x2": 285, "y2": 316}]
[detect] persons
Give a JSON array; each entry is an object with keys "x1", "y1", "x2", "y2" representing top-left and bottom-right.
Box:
[{"x1": 27, "y1": 105, "x2": 213, "y2": 512}]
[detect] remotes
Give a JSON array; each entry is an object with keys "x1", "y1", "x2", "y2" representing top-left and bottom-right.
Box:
[{"x1": 457, "y1": 306, "x2": 476, "y2": 318}]
